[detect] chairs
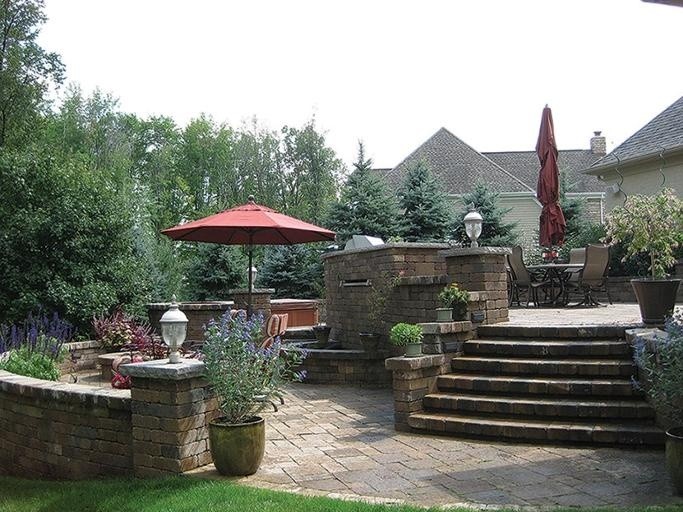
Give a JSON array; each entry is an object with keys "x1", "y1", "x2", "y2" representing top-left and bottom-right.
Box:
[
  {"x1": 505, "y1": 243, "x2": 612, "y2": 309},
  {"x1": 227, "y1": 309, "x2": 281, "y2": 412}
]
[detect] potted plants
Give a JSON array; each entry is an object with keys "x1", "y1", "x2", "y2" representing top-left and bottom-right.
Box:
[
  {"x1": 436, "y1": 287, "x2": 468, "y2": 321},
  {"x1": 603, "y1": 187, "x2": 683, "y2": 323},
  {"x1": 359, "y1": 271, "x2": 403, "y2": 351},
  {"x1": 389, "y1": 322, "x2": 424, "y2": 357}
]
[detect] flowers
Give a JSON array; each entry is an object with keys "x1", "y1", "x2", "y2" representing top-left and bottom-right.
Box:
[
  {"x1": 631, "y1": 307, "x2": 683, "y2": 427},
  {"x1": 197, "y1": 307, "x2": 309, "y2": 424}
]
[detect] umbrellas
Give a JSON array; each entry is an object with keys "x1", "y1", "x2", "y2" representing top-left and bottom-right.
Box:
[
  {"x1": 533, "y1": 103, "x2": 568, "y2": 301},
  {"x1": 159, "y1": 192, "x2": 337, "y2": 320}
]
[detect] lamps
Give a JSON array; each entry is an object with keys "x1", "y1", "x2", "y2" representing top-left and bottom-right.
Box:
[
  {"x1": 159, "y1": 294, "x2": 188, "y2": 364},
  {"x1": 245, "y1": 264, "x2": 257, "y2": 289},
  {"x1": 464, "y1": 202, "x2": 483, "y2": 247}
]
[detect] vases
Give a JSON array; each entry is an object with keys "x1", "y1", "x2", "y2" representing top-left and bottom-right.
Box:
[
  {"x1": 664, "y1": 427, "x2": 683, "y2": 496},
  {"x1": 208, "y1": 417, "x2": 265, "y2": 477},
  {"x1": 311, "y1": 327, "x2": 330, "y2": 344}
]
